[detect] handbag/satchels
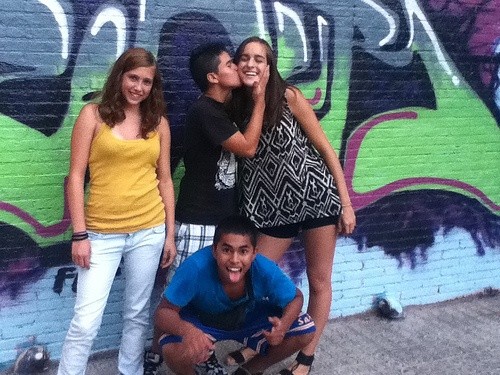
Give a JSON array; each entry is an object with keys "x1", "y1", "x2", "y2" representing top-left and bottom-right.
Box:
[{"x1": 199, "y1": 304, "x2": 246, "y2": 331}]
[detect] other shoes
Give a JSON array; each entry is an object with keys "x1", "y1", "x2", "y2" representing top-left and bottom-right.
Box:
[{"x1": 232, "y1": 367, "x2": 263, "y2": 375}]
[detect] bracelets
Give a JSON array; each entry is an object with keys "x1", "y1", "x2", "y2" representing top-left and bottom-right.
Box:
[
  {"x1": 71, "y1": 231, "x2": 89, "y2": 241},
  {"x1": 342, "y1": 203, "x2": 352, "y2": 207}
]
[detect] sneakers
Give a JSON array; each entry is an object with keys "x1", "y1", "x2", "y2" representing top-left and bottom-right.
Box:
[
  {"x1": 144, "y1": 350, "x2": 163, "y2": 375},
  {"x1": 196, "y1": 350, "x2": 230, "y2": 375}
]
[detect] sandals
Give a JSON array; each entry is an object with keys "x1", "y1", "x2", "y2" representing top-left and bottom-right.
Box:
[
  {"x1": 224, "y1": 346, "x2": 258, "y2": 366},
  {"x1": 279, "y1": 351, "x2": 314, "y2": 375}
]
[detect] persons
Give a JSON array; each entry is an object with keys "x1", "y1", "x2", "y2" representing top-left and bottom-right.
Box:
[
  {"x1": 225, "y1": 37, "x2": 357, "y2": 375},
  {"x1": 142, "y1": 43, "x2": 270, "y2": 375},
  {"x1": 153, "y1": 214, "x2": 316, "y2": 375},
  {"x1": 57, "y1": 48, "x2": 178, "y2": 375}
]
